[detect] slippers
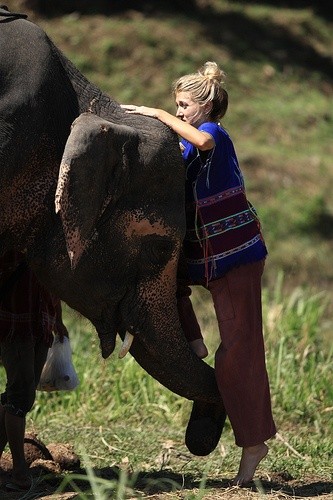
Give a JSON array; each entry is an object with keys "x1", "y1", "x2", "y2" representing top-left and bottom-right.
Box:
[{"x1": 5, "y1": 483, "x2": 28, "y2": 492}]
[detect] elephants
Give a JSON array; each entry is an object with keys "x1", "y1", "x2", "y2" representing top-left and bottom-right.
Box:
[{"x1": 0, "y1": 4, "x2": 227, "y2": 500}]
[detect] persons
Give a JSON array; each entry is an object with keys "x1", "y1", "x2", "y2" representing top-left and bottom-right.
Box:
[
  {"x1": 119, "y1": 60, "x2": 277, "y2": 485},
  {"x1": 0, "y1": 259, "x2": 80, "y2": 492}
]
